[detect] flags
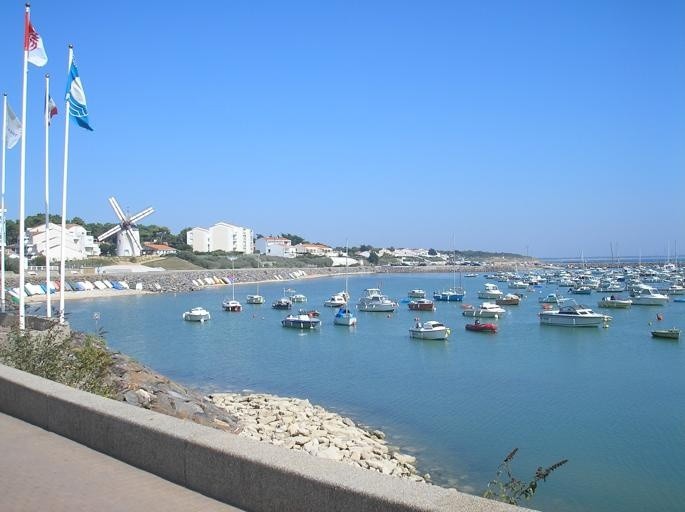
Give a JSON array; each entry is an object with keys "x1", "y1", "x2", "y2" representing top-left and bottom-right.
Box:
[
  {"x1": 45, "y1": 94, "x2": 58, "y2": 126},
  {"x1": 65, "y1": 54, "x2": 96, "y2": 132},
  {"x1": 5, "y1": 101, "x2": 21, "y2": 148},
  {"x1": 24, "y1": 11, "x2": 48, "y2": 66}
]
